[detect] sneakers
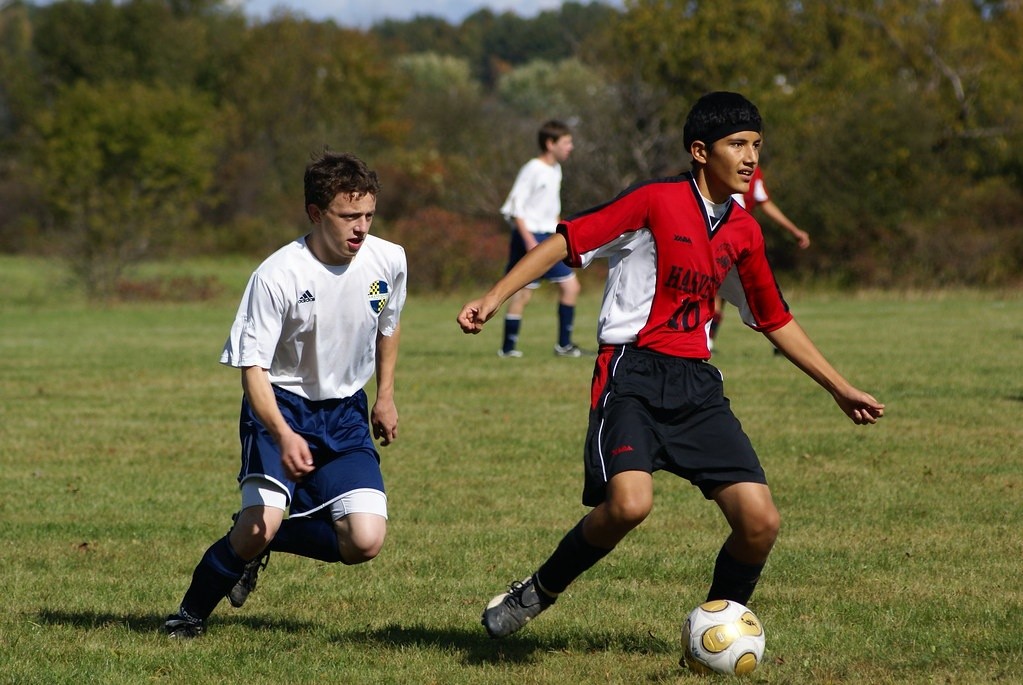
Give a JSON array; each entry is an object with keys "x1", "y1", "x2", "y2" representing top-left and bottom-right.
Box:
[
  {"x1": 226, "y1": 510, "x2": 272, "y2": 608},
  {"x1": 481, "y1": 576, "x2": 550, "y2": 640},
  {"x1": 166, "y1": 615, "x2": 205, "y2": 641}
]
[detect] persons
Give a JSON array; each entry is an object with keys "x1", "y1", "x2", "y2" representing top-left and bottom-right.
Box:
[
  {"x1": 163, "y1": 154, "x2": 408, "y2": 639},
  {"x1": 456, "y1": 91, "x2": 885, "y2": 671},
  {"x1": 497, "y1": 119, "x2": 583, "y2": 360}
]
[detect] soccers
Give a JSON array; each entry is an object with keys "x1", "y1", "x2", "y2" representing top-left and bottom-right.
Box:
[{"x1": 678, "y1": 597, "x2": 768, "y2": 681}]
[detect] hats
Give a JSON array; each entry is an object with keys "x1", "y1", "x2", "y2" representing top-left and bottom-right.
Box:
[{"x1": 684, "y1": 91, "x2": 762, "y2": 154}]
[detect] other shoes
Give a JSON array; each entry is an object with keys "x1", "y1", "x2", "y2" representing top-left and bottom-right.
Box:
[
  {"x1": 554, "y1": 343, "x2": 584, "y2": 358},
  {"x1": 497, "y1": 349, "x2": 521, "y2": 358}
]
